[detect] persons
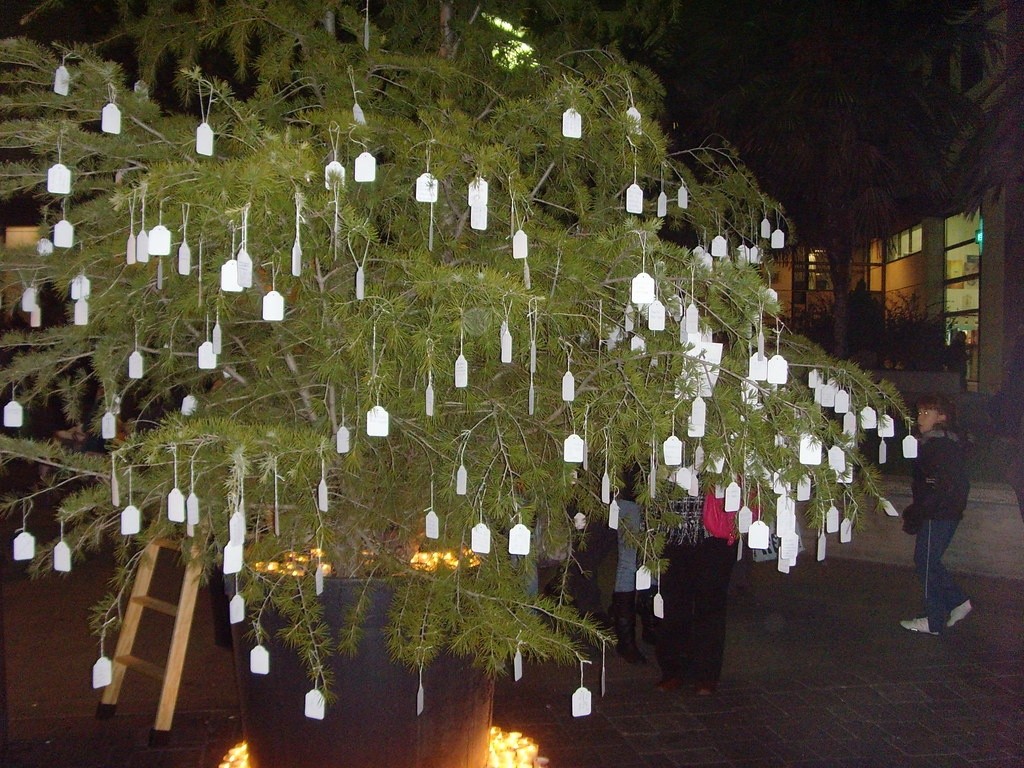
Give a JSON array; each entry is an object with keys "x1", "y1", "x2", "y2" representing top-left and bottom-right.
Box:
[
  {"x1": 950, "y1": 331, "x2": 968, "y2": 390},
  {"x1": 610, "y1": 464, "x2": 663, "y2": 665},
  {"x1": 638, "y1": 467, "x2": 762, "y2": 696},
  {"x1": 900, "y1": 390, "x2": 974, "y2": 636},
  {"x1": 563, "y1": 504, "x2": 612, "y2": 618}
]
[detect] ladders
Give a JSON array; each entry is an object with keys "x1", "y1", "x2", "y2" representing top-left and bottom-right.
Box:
[{"x1": 96, "y1": 500, "x2": 217, "y2": 747}]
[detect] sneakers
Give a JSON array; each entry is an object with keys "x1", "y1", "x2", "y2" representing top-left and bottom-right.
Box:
[
  {"x1": 899, "y1": 615, "x2": 940, "y2": 636},
  {"x1": 946, "y1": 600, "x2": 972, "y2": 628}
]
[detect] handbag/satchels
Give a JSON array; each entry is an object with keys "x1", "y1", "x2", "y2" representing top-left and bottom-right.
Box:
[{"x1": 902, "y1": 504, "x2": 922, "y2": 534}]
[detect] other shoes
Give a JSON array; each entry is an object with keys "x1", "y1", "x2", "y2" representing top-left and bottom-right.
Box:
[
  {"x1": 653, "y1": 675, "x2": 679, "y2": 692},
  {"x1": 696, "y1": 677, "x2": 715, "y2": 696}
]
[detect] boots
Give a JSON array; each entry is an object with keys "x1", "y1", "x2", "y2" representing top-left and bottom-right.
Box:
[
  {"x1": 612, "y1": 590, "x2": 644, "y2": 665},
  {"x1": 640, "y1": 585, "x2": 663, "y2": 645}
]
